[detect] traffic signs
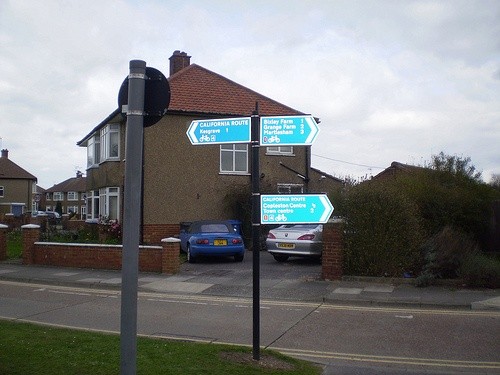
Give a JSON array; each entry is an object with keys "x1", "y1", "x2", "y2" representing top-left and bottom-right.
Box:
[
  {"x1": 259, "y1": 114, "x2": 320, "y2": 147},
  {"x1": 186, "y1": 116, "x2": 252, "y2": 146},
  {"x1": 260, "y1": 192, "x2": 335, "y2": 224}
]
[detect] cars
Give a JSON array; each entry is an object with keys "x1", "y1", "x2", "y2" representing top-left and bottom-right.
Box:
[
  {"x1": 266, "y1": 224, "x2": 324, "y2": 263},
  {"x1": 21, "y1": 210, "x2": 62, "y2": 220},
  {"x1": 179, "y1": 220, "x2": 245, "y2": 264}
]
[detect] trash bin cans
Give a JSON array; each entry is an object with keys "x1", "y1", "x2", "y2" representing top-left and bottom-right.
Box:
[{"x1": 224, "y1": 220, "x2": 242, "y2": 238}]
